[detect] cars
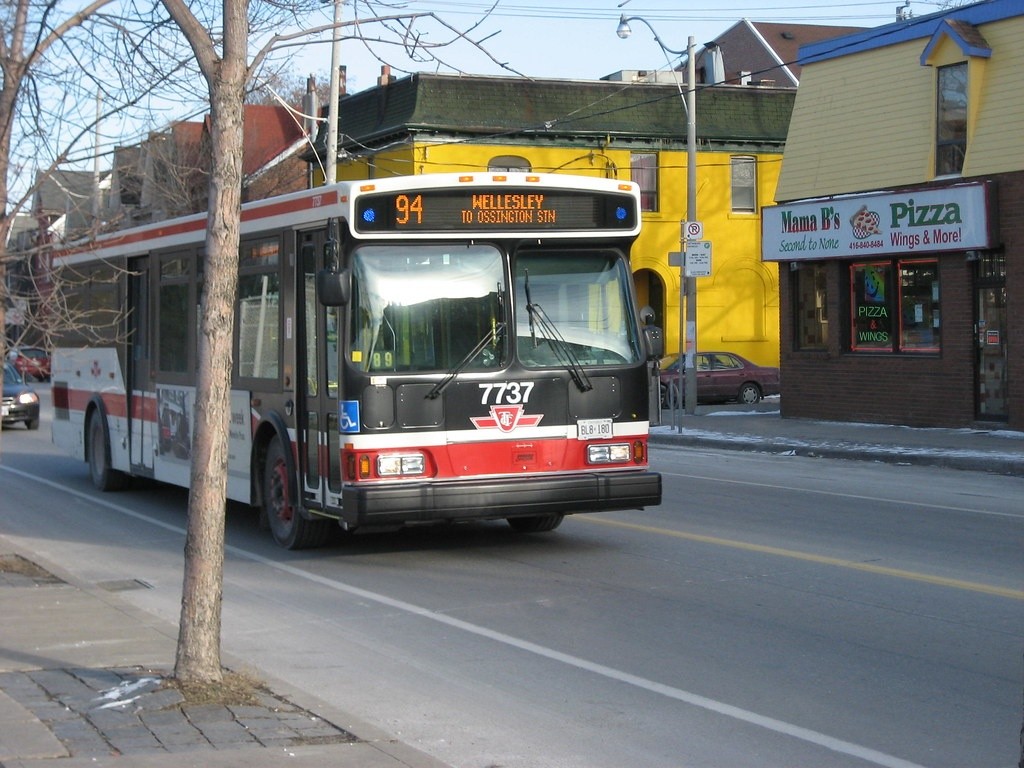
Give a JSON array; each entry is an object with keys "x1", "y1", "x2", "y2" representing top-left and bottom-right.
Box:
[
  {"x1": 4, "y1": 346, "x2": 52, "y2": 382},
  {"x1": 659, "y1": 351, "x2": 782, "y2": 410},
  {"x1": 1, "y1": 360, "x2": 41, "y2": 432}
]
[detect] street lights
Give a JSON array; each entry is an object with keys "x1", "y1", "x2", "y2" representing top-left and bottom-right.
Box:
[{"x1": 616, "y1": 15, "x2": 697, "y2": 412}]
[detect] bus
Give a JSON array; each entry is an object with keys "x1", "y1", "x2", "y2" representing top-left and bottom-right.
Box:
[{"x1": 52, "y1": 171, "x2": 667, "y2": 556}]
